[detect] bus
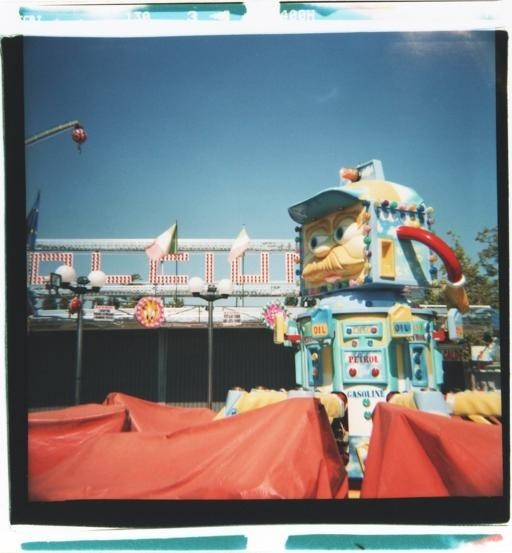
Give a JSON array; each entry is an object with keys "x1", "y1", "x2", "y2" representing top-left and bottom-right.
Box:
[{"x1": 418, "y1": 300, "x2": 497, "y2": 365}]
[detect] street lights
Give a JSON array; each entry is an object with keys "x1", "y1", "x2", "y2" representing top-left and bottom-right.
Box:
[
  {"x1": 44, "y1": 265, "x2": 108, "y2": 406},
  {"x1": 189, "y1": 284, "x2": 229, "y2": 411}
]
